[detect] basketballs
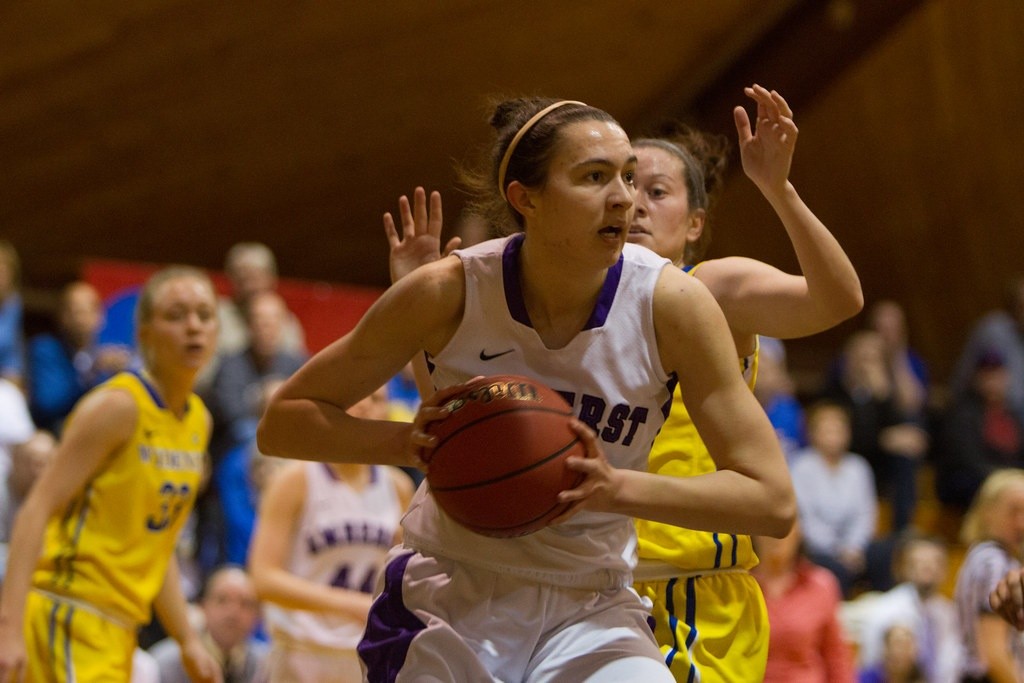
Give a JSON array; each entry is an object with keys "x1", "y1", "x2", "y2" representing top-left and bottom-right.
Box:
[{"x1": 417, "y1": 375, "x2": 588, "y2": 542}]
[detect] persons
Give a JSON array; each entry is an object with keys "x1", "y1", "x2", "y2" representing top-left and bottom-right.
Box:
[
  {"x1": 383, "y1": 80, "x2": 868, "y2": 683},
  {"x1": 0, "y1": 241, "x2": 1024, "y2": 683},
  {"x1": 1, "y1": 264, "x2": 219, "y2": 683},
  {"x1": 257, "y1": 97, "x2": 804, "y2": 682}
]
